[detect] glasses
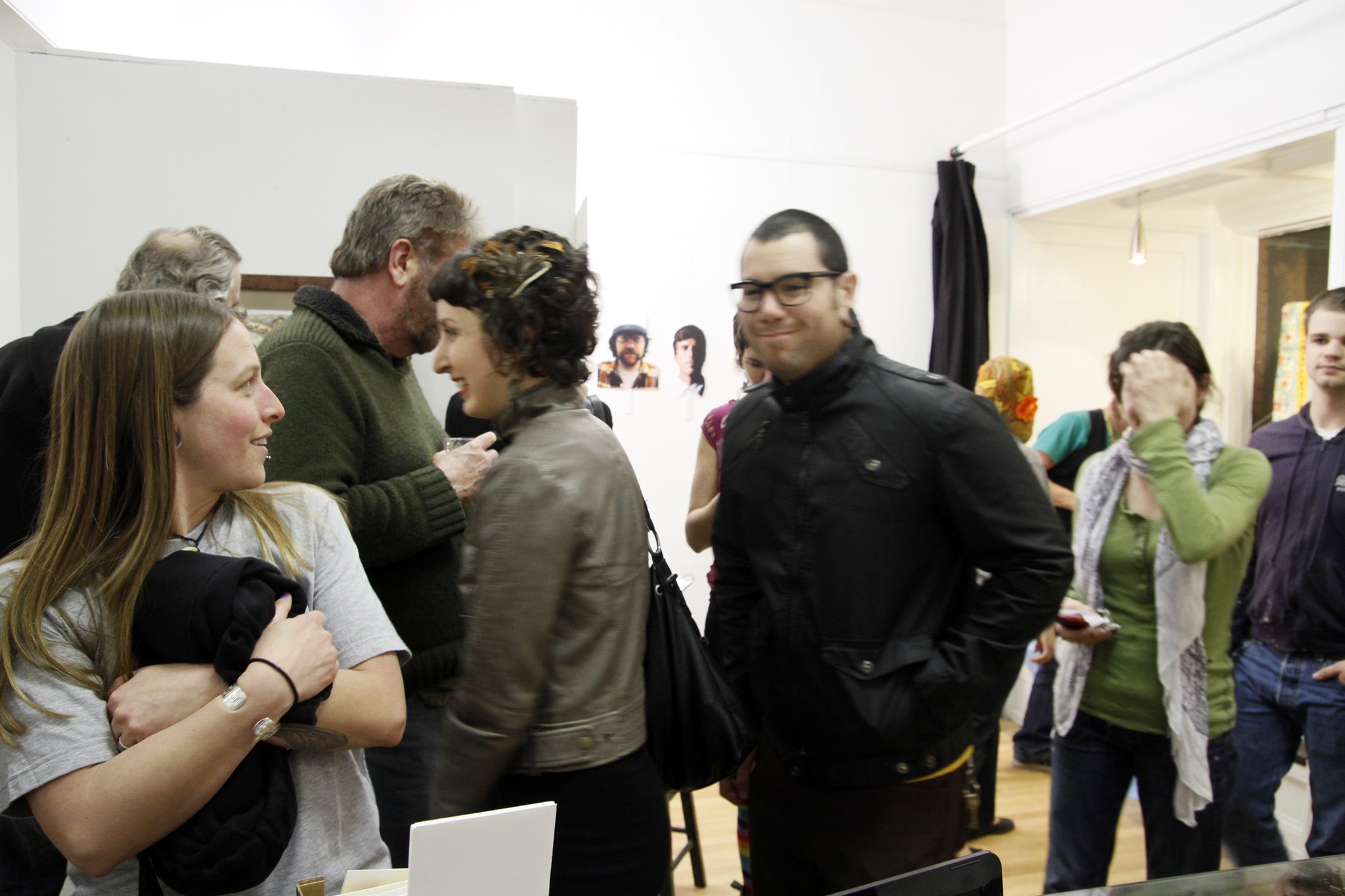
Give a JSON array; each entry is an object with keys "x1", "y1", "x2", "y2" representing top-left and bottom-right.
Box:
[
  {"x1": 225, "y1": 304, "x2": 248, "y2": 320},
  {"x1": 729, "y1": 271, "x2": 843, "y2": 315}
]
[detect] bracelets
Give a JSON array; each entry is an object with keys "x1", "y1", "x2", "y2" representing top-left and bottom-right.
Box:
[
  {"x1": 223, "y1": 684, "x2": 282, "y2": 745},
  {"x1": 240, "y1": 656, "x2": 302, "y2": 711}
]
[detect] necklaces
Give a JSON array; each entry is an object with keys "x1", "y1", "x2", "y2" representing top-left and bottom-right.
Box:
[{"x1": 169, "y1": 517, "x2": 214, "y2": 556}]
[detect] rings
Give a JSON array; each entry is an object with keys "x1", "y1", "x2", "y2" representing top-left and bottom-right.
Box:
[{"x1": 116, "y1": 736, "x2": 131, "y2": 751}]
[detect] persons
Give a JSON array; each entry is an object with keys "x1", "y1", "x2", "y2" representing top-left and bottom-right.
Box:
[
  {"x1": 1024, "y1": 333, "x2": 1135, "y2": 764},
  {"x1": 0, "y1": 212, "x2": 247, "y2": 539},
  {"x1": 672, "y1": 321, "x2": 712, "y2": 394},
  {"x1": 1214, "y1": 279, "x2": 1345, "y2": 870},
  {"x1": 409, "y1": 222, "x2": 671, "y2": 896},
  {"x1": 696, "y1": 209, "x2": 1080, "y2": 896},
  {"x1": 242, "y1": 169, "x2": 508, "y2": 844},
  {"x1": 598, "y1": 320, "x2": 660, "y2": 389},
  {"x1": 683, "y1": 306, "x2": 776, "y2": 600},
  {"x1": 1026, "y1": 318, "x2": 1280, "y2": 896},
  {"x1": 0, "y1": 283, "x2": 413, "y2": 896}
]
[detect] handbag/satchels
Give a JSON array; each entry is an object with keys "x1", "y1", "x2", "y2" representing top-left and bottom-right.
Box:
[{"x1": 646, "y1": 551, "x2": 752, "y2": 795}]
